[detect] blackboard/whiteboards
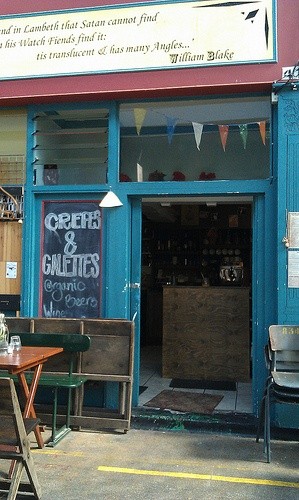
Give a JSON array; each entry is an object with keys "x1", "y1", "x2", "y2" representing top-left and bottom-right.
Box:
[{"x1": 38, "y1": 199, "x2": 104, "y2": 318}]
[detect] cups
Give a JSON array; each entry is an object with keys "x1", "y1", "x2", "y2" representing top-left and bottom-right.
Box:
[{"x1": 10, "y1": 336, "x2": 22, "y2": 352}]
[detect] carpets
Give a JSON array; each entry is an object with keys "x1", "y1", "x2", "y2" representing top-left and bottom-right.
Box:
[{"x1": 142, "y1": 389, "x2": 224, "y2": 415}]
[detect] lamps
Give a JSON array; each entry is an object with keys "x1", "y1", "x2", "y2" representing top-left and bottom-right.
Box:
[{"x1": 98, "y1": 190, "x2": 124, "y2": 208}]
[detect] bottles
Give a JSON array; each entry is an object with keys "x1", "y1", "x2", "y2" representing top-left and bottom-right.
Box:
[
  {"x1": 0, "y1": 313, "x2": 9, "y2": 355},
  {"x1": 142, "y1": 223, "x2": 240, "y2": 269},
  {"x1": 7, "y1": 343, "x2": 13, "y2": 354}
]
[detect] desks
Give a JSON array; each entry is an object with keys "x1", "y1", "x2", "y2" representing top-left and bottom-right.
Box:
[{"x1": 0, "y1": 338, "x2": 63, "y2": 472}]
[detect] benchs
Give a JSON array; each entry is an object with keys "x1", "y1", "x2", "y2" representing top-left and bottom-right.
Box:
[
  {"x1": 0, "y1": 374, "x2": 45, "y2": 500},
  {"x1": 4, "y1": 313, "x2": 138, "y2": 433}
]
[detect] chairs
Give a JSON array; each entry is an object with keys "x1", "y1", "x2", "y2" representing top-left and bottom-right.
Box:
[{"x1": 257, "y1": 312, "x2": 299, "y2": 463}]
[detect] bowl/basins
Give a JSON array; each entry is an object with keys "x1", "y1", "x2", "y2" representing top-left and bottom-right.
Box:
[{"x1": 219, "y1": 265, "x2": 243, "y2": 286}]
